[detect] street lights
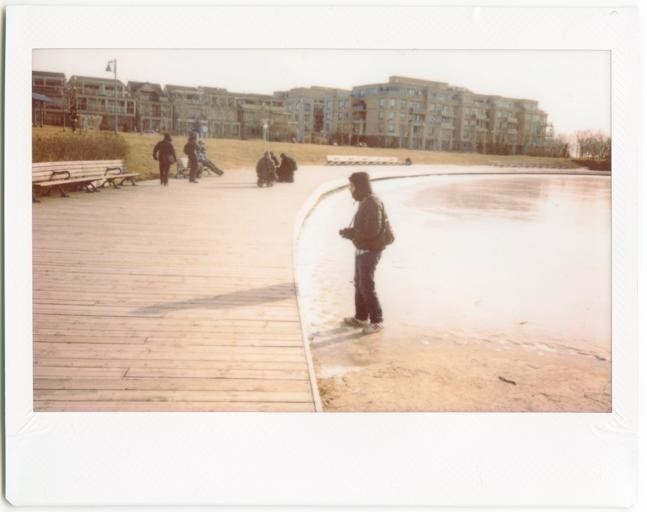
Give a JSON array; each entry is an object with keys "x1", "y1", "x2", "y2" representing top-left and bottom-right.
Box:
[{"x1": 105, "y1": 58, "x2": 118, "y2": 134}]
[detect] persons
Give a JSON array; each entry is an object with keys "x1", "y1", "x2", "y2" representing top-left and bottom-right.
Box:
[
  {"x1": 277, "y1": 153, "x2": 297, "y2": 183},
  {"x1": 69, "y1": 110, "x2": 79, "y2": 133},
  {"x1": 183, "y1": 131, "x2": 224, "y2": 183},
  {"x1": 152, "y1": 132, "x2": 175, "y2": 185},
  {"x1": 270, "y1": 152, "x2": 279, "y2": 165},
  {"x1": 338, "y1": 170, "x2": 394, "y2": 333},
  {"x1": 255, "y1": 152, "x2": 275, "y2": 186}
]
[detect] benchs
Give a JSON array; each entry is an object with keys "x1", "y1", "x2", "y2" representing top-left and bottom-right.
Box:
[{"x1": 32, "y1": 159, "x2": 142, "y2": 203}]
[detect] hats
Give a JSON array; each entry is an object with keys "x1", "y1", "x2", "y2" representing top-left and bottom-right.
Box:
[{"x1": 349, "y1": 173, "x2": 372, "y2": 201}]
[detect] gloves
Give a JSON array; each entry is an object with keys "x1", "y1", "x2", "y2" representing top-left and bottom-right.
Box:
[{"x1": 339, "y1": 228, "x2": 357, "y2": 238}]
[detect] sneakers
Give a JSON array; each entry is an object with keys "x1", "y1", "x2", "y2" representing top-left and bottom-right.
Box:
[{"x1": 344, "y1": 317, "x2": 384, "y2": 334}]
